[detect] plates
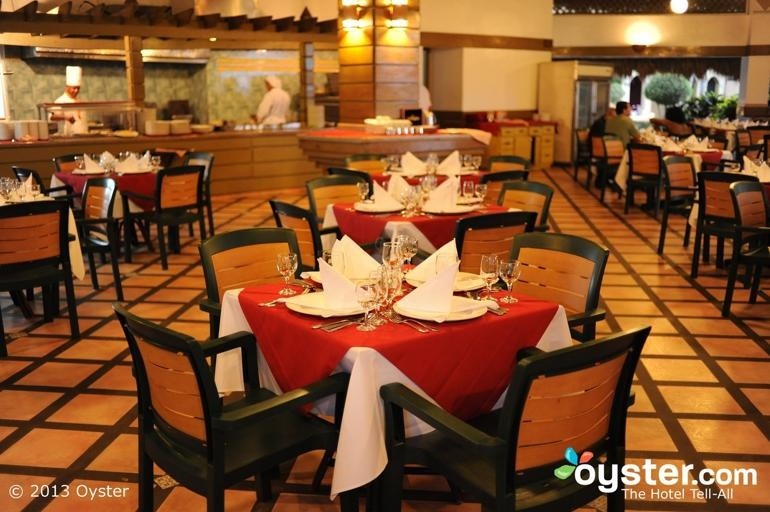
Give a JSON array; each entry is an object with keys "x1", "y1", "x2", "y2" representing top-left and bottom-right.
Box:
[
  {"x1": 354, "y1": 202, "x2": 404, "y2": 212},
  {"x1": 392, "y1": 296, "x2": 488, "y2": 321},
  {"x1": 137, "y1": 108, "x2": 214, "y2": 136},
  {"x1": 285, "y1": 293, "x2": 376, "y2": 317},
  {"x1": 422, "y1": 205, "x2": 477, "y2": 214},
  {"x1": 1, "y1": 120, "x2": 49, "y2": 141},
  {"x1": 406, "y1": 272, "x2": 486, "y2": 292}
]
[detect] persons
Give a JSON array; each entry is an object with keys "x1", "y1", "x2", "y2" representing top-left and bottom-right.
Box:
[
  {"x1": 249, "y1": 76, "x2": 291, "y2": 129},
  {"x1": 422, "y1": 85, "x2": 434, "y2": 112},
  {"x1": 604, "y1": 102, "x2": 642, "y2": 193},
  {"x1": 584, "y1": 106, "x2": 617, "y2": 188},
  {"x1": 48, "y1": 83, "x2": 93, "y2": 134},
  {"x1": 647, "y1": 107, "x2": 695, "y2": 142}
]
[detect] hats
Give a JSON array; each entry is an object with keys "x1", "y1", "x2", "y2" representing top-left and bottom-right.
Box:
[
  {"x1": 66, "y1": 66, "x2": 82, "y2": 87},
  {"x1": 265, "y1": 76, "x2": 282, "y2": 88}
]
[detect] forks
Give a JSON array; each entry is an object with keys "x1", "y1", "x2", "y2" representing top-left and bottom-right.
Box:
[
  {"x1": 388, "y1": 311, "x2": 439, "y2": 332},
  {"x1": 266, "y1": 287, "x2": 310, "y2": 308}
]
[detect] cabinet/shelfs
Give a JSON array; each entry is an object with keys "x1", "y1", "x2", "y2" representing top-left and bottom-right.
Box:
[{"x1": 482, "y1": 125, "x2": 555, "y2": 170}]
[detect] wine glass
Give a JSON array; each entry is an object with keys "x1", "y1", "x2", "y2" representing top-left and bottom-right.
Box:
[
  {"x1": 399, "y1": 176, "x2": 438, "y2": 218},
  {"x1": 381, "y1": 151, "x2": 483, "y2": 175},
  {"x1": 275, "y1": 252, "x2": 299, "y2": 295},
  {"x1": 479, "y1": 255, "x2": 501, "y2": 303},
  {"x1": 355, "y1": 234, "x2": 419, "y2": 333},
  {"x1": 671, "y1": 134, "x2": 702, "y2": 150},
  {"x1": 476, "y1": 184, "x2": 488, "y2": 208},
  {"x1": 74, "y1": 150, "x2": 162, "y2": 173},
  {"x1": 499, "y1": 259, "x2": 521, "y2": 304},
  {"x1": 0, "y1": 176, "x2": 21, "y2": 200},
  {"x1": 357, "y1": 180, "x2": 369, "y2": 201},
  {"x1": 464, "y1": 180, "x2": 475, "y2": 207},
  {"x1": 731, "y1": 158, "x2": 763, "y2": 181}
]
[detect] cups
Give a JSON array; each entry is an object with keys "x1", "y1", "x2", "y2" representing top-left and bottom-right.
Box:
[{"x1": 384, "y1": 127, "x2": 425, "y2": 135}]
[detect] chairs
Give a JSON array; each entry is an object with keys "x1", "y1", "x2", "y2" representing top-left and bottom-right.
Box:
[{"x1": 572, "y1": 126, "x2": 770, "y2": 319}]
